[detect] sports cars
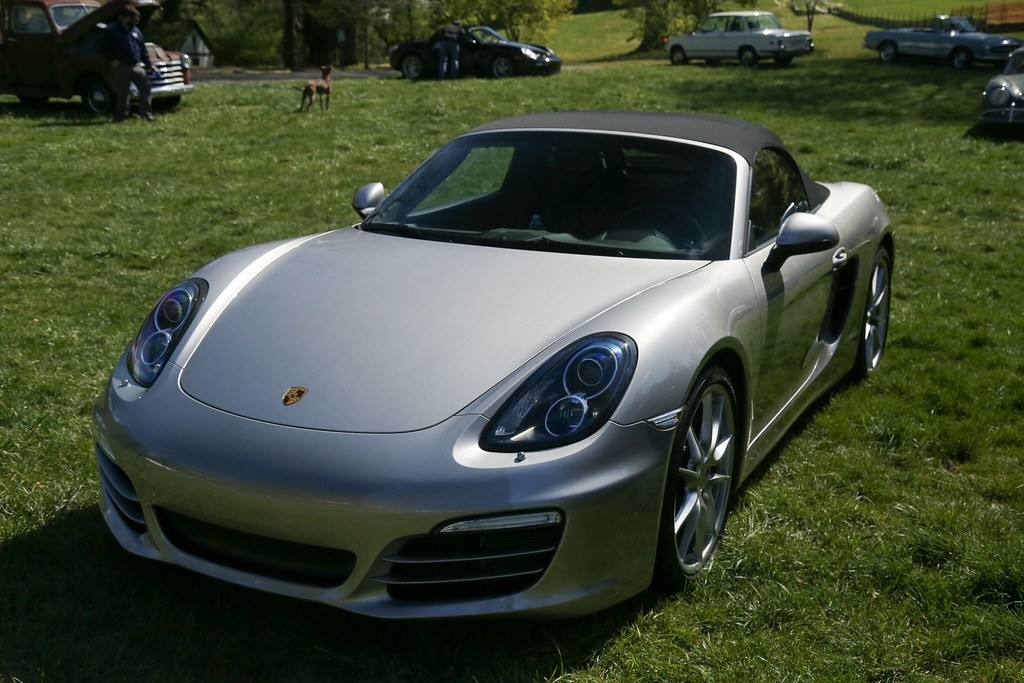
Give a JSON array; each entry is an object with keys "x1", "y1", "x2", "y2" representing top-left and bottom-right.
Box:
[{"x1": 91, "y1": 106, "x2": 896, "y2": 624}]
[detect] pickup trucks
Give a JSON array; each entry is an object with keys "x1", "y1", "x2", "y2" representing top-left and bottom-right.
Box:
[{"x1": 862, "y1": 13, "x2": 1021, "y2": 70}]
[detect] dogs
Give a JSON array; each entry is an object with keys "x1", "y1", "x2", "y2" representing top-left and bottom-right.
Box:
[{"x1": 293, "y1": 64, "x2": 332, "y2": 113}]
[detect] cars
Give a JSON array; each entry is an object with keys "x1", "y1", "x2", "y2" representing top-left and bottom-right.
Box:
[
  {"x1": 979, "y1": 46, "x2": 1024, "y2": 127},
  {"x1": 389, "y1": 24, "x2": 563, "y2": 81},
  {"x1": 0, "y1": 1, "x2": 196, "y2": 116},
  {"x1": 663, "y1": 10, "x2": 815, "y2": 68}
]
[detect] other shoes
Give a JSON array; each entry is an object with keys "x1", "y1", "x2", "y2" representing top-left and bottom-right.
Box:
[
  {"x1": 117, "y1": 114, "x2": 126, "y2": 122},
  {"x1": 451, "y1": 75, "x2": 455, "y2": 79},
  {"x1": 440, "y1": 76, "x2": 445, "y2": 79},
  {"x1": 144, "y1": 111, "x2": 154, "y2": 121}
]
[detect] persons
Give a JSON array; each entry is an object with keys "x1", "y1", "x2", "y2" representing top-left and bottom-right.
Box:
[
  {"x1": 94, "y1": 5, "x2": 158, "y2": 121},
  {"x1": 438, "y1": 21, "x2": 472, "y2": 81}
]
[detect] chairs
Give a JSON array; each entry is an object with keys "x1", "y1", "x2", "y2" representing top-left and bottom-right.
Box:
[
  {"x1": 730, "y1": 22, "x2": 740, "y2": 32},
  {"x1": 747, "y1": 22, "x2": 754, "y2": 31}
]
